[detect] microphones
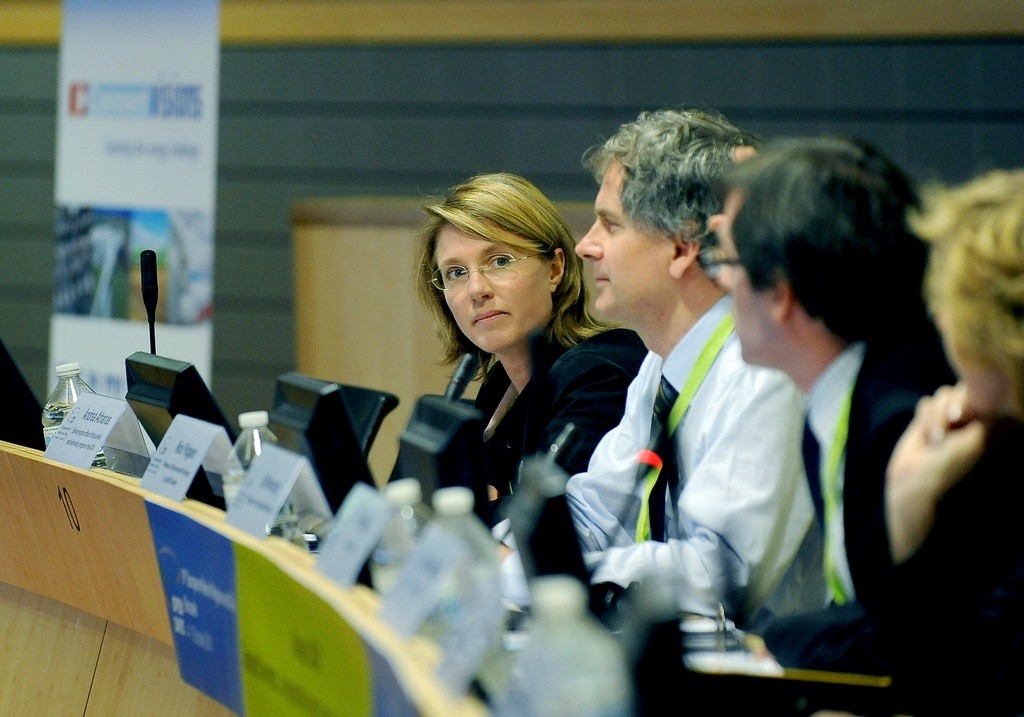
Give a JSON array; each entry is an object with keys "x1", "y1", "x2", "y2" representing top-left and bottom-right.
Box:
[{"x1": 141, "y1": 250, "x2": 159, "y2": 354}]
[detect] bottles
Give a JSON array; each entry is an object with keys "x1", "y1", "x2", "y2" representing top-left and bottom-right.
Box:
[
  {"x1": 414, "y1": 486, "x2": 506, "y2": 696},
  {"x1": 222, "y1": 410, "x2": 311, "y2": 553},
  {"x1": 491, "y1": 575, "x2": 633, "y2": 717},
  {"x1": 38, "y1": 363, "x2": 112, "y2": 472},
  {"x1": 371, "y1": 479, "x2": 436, "y2": 597}
]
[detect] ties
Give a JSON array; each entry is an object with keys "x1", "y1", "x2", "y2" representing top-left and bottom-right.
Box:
[
  {"x1": 642, "y1": 378, "x2": 679, "y2": 542},
  {"x1": 799, "y1": 415, "x2": 830, "y2": 538}
]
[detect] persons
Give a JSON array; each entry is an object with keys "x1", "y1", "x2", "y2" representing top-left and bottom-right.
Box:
[
  {"x1": 489, "y1": 108, "x2": 846, "y2": 673},
  {"x1": 699, "y1": 125, "x2": 963, "y2": 678},
  {"x1": 842, "y1": 162, "x2": 1024, "y2": 717},
  {"x1": 414, "y1": 173, "x2": 652, "y2": 621}
]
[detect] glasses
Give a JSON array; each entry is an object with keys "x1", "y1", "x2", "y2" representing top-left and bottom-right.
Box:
[
  {"x1": 428, "y1": 252, "x2": 546, "y2": 293},
  {"x1": 697, "y1": 247, "x2": 746, "y2": 282}
]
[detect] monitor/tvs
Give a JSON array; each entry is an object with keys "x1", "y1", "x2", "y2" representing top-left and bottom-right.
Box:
[
  {"x1": 119, "y1": 354, "x2": 237, "y2": 460},
  {"x1": 398, "y1": 394, "x2": 494, "y2": 526},
  {"x1": 271, "y1": 376, "x2": 375, "y2": 524},
  {"x1": 505, "y1": 456, "x2": 601, "y2": 615}
]
